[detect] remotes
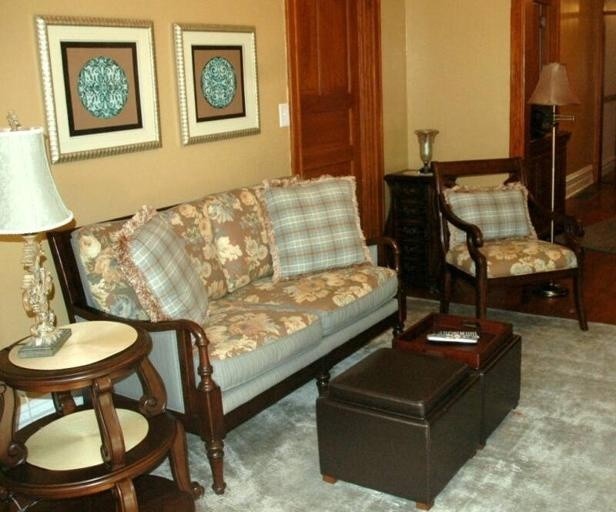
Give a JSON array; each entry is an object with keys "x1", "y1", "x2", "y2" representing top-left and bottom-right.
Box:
[{"x1": 426, "y1": 330, "x2": 481, "y2": 345}]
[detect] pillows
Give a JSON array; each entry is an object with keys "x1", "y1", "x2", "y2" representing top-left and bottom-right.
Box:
[
  {"x1": 112, "y1": 205, "x2": 214, "y2": 339},
  {"x1": 253, "y1": 173, "x2": 375, "y2": 285},
  {"x1": 439, "y1": 183, "x2": 534, "y2": 252}
]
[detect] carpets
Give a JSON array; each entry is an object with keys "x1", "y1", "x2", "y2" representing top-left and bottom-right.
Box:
[
  {"x1": 153, "y1": 289, "x2": 614, "y2": 511},
  {"x1": 554, "y1": 215, "x2": 615, "y2": 254}
]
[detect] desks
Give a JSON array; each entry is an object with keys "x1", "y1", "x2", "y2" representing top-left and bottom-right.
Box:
[{"x1": 0, "y1": 318, "x2": 206, "y2": 512}]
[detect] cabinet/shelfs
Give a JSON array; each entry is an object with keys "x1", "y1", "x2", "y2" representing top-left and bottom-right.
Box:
[
  {"x1": 533, "y1": 128, "x2": 585, "y2": 240},
  {"x1": 383, "y1": 165, "x2": 447, "y2": 286}
]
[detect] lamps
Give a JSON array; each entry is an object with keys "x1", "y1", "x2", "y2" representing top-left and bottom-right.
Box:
[
  {"x1": 1, "y1": 118, "x2": 79, "y2": 361},
  {"x1": 528, "y1": 62, "x2": 583, "y2": 300}
]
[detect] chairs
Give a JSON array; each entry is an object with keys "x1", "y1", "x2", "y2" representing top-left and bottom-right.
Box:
[{"x1": 429, "y1": 156, "x2": 592, "y2": 336}]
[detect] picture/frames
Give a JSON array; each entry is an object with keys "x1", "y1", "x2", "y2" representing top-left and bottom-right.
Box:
[
  {"x1": 171, "y1": 18, "x2": 261, "y2": 151},
  {"x1": 31, "y1": 11, "x2": 167, "y2": 167}
]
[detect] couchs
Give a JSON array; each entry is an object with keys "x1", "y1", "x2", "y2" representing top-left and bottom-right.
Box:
[{"x1": 45, "y1": 171, "x2": 403, "y2": 497}]
